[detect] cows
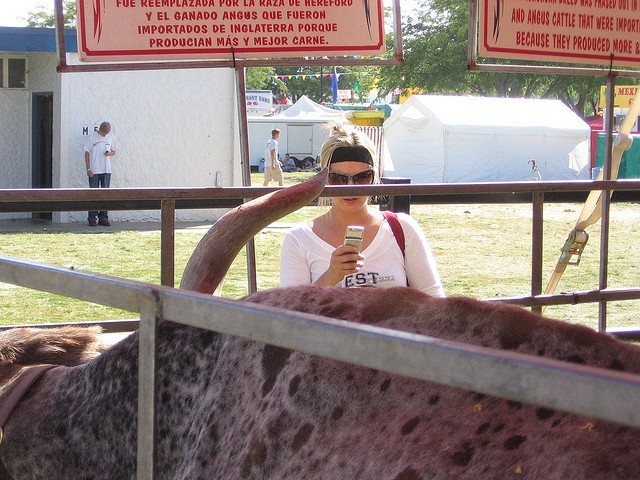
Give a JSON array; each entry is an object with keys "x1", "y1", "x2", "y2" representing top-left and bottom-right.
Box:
[{"x1": 1, "y1": 167, "x2": 640, "y2": 480}]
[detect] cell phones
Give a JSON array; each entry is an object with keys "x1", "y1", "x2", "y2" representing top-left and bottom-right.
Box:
[{"x1": 344, "y1": 226, "x2": 366, "y2": 252}]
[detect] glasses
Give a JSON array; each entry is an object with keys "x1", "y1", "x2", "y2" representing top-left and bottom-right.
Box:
[{"x1": 328, "y1": 170, "x2": 375, "y2": 184}]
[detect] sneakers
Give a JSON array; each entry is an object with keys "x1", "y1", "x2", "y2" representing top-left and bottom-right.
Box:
[
  {"x1": 99, "y1": 219, "x2": 111, "y2": 226},
  {"x1": 89, "y1": 220, "x2": 96, "y2": 226}
]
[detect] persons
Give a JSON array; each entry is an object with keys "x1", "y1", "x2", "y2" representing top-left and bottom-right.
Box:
[
  {"x1": 263, "y1": 128, "x2": 284, "y2": 186},
  {"x1": 280, "y1": 125, "x2": 446, "y2": 299},
  {"x1": 283, "y1": 153, "x2": 298, "y2": 172},
  {"x1": 85, "y1": 121, "x2": 116, "y2": 226}
]
